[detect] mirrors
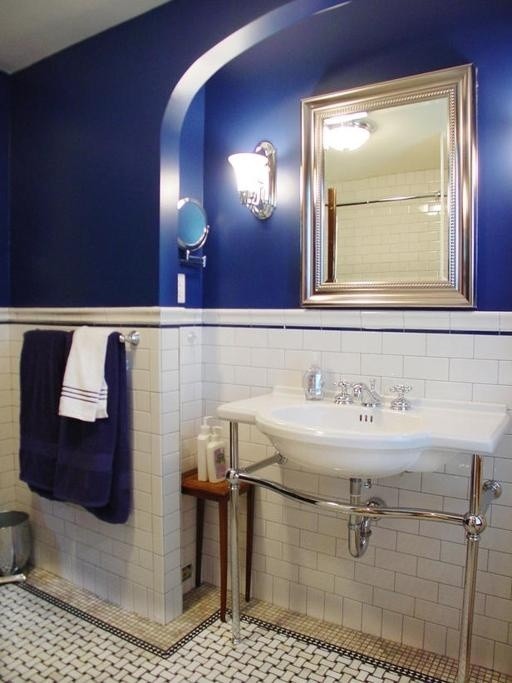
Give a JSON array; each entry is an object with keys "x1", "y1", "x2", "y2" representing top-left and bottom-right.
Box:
[
  {"x1": 178, "y1": 198, "x2": 209, "y2": 268},
  {"x1": 298, "y1": 62, "x2": 478, "y2": 309}
]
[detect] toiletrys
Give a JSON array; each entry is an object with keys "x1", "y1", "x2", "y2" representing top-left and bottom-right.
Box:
[
  {"x1": 196, "y1": 414, "x2": 227, "y2": 484},
  {"x1": 302, "y1": 360, "x2": 325, "y2": 400}
]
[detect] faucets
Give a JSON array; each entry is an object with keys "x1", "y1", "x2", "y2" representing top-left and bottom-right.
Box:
[{"x1": 352, "y1": 384, "x2": 380, "y2": 407}]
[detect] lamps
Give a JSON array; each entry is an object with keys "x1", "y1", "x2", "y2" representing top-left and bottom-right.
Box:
[
  {"x1": 226, "y1": 139, "x2": 275, "y2": 220},
  {"x1": 321, "y1": 112, "x2": 372, "y2": 151}
]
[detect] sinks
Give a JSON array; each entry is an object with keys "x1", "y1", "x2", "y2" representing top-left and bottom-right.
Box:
[{"x1": 219, "y1": 388, "x2": 510, "y2": 480}]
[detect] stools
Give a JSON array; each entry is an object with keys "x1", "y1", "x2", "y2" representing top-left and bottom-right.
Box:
[{"x1": 181, "y1": 465, "x2": 254, "y2": 622}]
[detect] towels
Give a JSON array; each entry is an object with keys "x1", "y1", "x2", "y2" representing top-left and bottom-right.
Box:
[
  {"x1": 18, "y1": 328, "x2": 132, "y2": 525},
  {"x1": 58, "y1": 325, "x2": 121, "y2": 422}
]
